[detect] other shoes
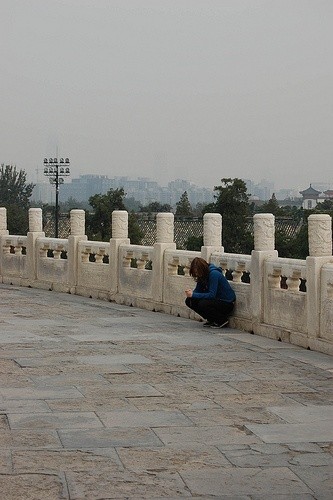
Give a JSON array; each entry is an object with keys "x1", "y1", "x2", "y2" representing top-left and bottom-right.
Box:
[
  {"x1": 203, "y1": 321, "x2": 215, "y2": 327},
  {"x1": 210, "y1": 320, "x2": 229, "y2": 329}
]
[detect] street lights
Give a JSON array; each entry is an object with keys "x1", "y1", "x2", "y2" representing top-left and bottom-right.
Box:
[{"x1": 42, "y1": 157, "x2": 70, "y2": 238}]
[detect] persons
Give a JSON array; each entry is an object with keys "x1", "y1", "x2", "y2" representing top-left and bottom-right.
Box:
[{"x1": 184, "y1": 257, "x2": 236, "y2": 328}]
